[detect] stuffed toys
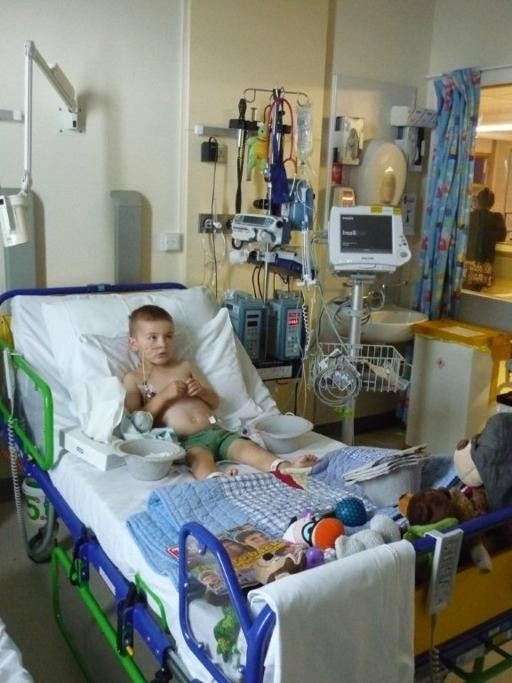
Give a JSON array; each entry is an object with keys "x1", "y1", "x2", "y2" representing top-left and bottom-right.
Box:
[
  {"x1": 262, "y1": 410, "x2": 512, "y2": 586},
  {"x1": 244, "y1": 125, "x2": 272, "y2": 181},
  {"x1": 261, "y1": 512, "x2": 402, "y2": 585},
  {"x1": 399, "y1": 411, "x2": 511, "y2": 575}
]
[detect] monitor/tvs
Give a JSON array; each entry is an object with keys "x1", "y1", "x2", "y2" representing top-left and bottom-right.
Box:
[{"x1": 331, "y1": 204, "x2": 410, "y2": 270}]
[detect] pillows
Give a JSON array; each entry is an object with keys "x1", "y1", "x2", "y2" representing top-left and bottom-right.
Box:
[{"x1": 44, "y1": 287, "x2": 264, "y2": 444}]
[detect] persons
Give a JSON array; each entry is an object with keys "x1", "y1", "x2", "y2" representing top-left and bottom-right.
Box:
[
  {"x1": 122, "y1": 304, "x2": 320, "y2": 484},
  {"x1": 464, "y1": 186, "x2": 507, "y2": 293},
  {"x1": 194, "y1": 527, "x2": 271, "y2": 592}
]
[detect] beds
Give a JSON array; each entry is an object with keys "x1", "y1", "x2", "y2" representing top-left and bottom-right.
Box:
[{"x1": 0, "y1": 281, "x2": 512, "y2": 682}]
